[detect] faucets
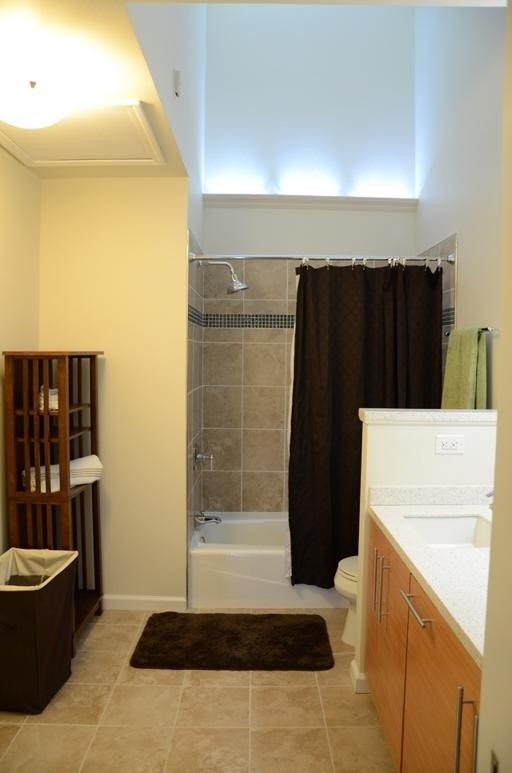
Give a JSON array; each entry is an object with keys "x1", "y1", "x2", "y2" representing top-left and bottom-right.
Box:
[{"x1": 193, "y1": 511, "x2": 223, "y2": 532}]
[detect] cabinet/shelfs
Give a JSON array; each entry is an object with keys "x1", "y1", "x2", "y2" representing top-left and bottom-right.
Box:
[
  {"x1": 2, "y1": 351, "x2": 105, "y2": 659},
  {"x1": 365, "y1": 517, "x2": 482, "y2": 771}
]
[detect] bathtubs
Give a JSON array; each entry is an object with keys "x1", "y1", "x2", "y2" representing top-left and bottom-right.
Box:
[{"x1": 189, "y1": 509, "x2": 345, "y2": 609}]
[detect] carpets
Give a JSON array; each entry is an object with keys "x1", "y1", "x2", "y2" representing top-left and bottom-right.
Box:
[{"x1": 130, "y1": 612, "x2": 335, "y2": 672}]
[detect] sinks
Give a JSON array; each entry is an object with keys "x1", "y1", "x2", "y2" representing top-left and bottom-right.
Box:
[{"x1": 402, "y1": 511, "x2": 492, "y2": 552}]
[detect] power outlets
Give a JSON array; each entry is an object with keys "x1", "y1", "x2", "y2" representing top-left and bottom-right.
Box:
[{"x1": 434, "y1": 433, "x2": 466, "y2": 455}]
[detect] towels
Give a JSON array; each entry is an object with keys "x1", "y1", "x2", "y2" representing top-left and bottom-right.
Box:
[
  {"x1": 39, "y1": 383, "x2": 59, "y2": 410},
  {"x1": 22, "y1": 455, "x2": 103, "y2": 494},
  {"x1": 440, "y1": 326, "x2": 489, "y2": 410}
]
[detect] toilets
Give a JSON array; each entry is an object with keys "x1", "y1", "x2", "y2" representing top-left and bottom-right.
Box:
[{"x1": 335, "y1": 554, "x2": 361, "y2": 644}]
